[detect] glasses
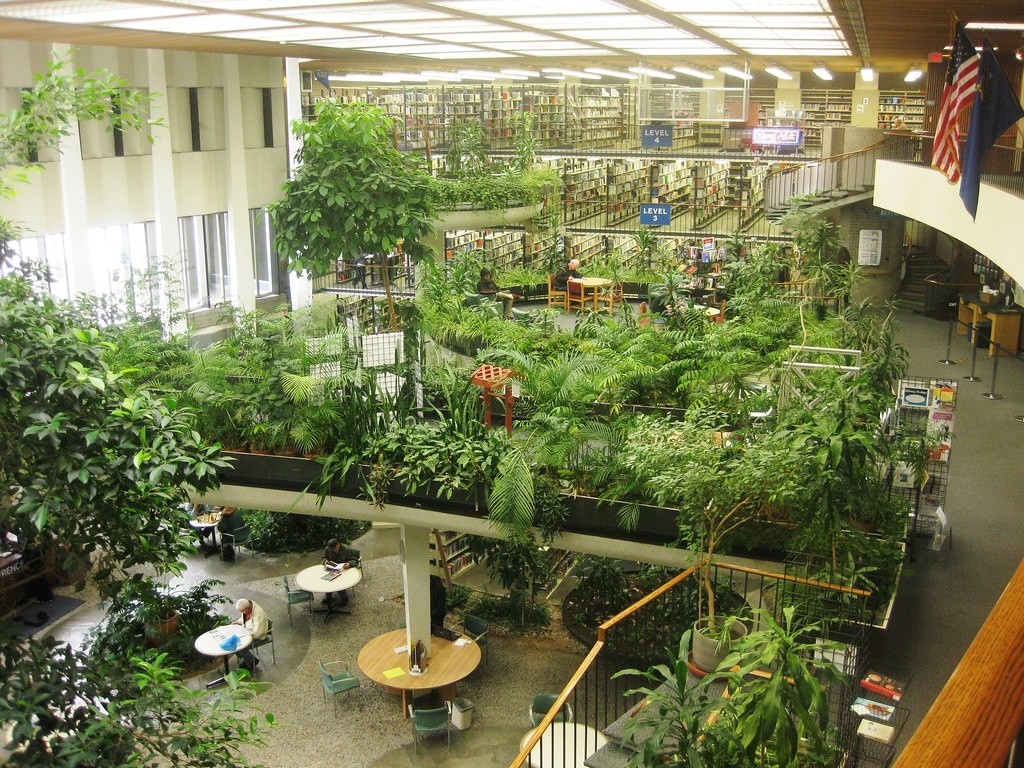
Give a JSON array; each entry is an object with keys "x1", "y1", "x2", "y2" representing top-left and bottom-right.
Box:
[{"x1": 240, "y1": 609, "x2": 246, "y2": 613}]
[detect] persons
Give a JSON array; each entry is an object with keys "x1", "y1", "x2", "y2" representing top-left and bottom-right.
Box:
[
  {"x1": 184, "y1": 493, "x2": 215, "y2": 547},
  {"x1": 649, "y1": 287, "x2": 682, "y2": 331},
  {"x1": 477, "y1": 267, "x2": 515, "y2": 319},
  {"x1": 231, "y1": 598, "x2": 269, "y2": 671},
  {"x1": 217, "y1": 505, "x2": 248, "y2": 562},
  {"x1": 350, "y1": 257, "x2": 368, "y2": 290},
  {"x1": 555, "y1": 259, "x2": 587, "y2": 310},
  {"x1": 892, "y1": 118, "x2": 909, "y2": 130},
  {"x1": 320, "y1": 539, "x2": 360, "y2": 608}
]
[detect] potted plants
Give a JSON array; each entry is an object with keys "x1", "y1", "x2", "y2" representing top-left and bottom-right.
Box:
[
  {"x1": 202, "y1": 390, "x2": 247, "y2": 452},
  {"x1": 267, "y1": 416, "x2": 299, "y2": 457},
  {"x1": 558, "y1": 475, "x2": 577, "y2": 493},
  {"x1": 271, "y1": 399, "x2": 344, "y2": 459},
  {"x1": 623, "y1": 407, "x2": 834, "y2": 674},
  {"x1": 747, "y1": 453, "x2": 809, "y2": 522},
  {"x1": 132, "y1": 574, "x2": 184, "y2": 637},
  {"x1": 425, "y1": 165, "x2": 567, "y2": 229},
  {"x1": 837, "y1": 482, "x2": 907, "y2": 533},
  {"x1": 237, "y1": 421, "x2": 272, "y2": 455},
  {"x1": 608, "y1": 606, "x2": 852, "y2": 768}
]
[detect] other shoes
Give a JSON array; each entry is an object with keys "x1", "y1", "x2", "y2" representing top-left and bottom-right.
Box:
[
  {"x1": 321, "y1": 593, "x2": 332, "y2": 605},
  {"x1": 340, "y1": 595, "x2": 348, "y2": 606},
  {"x1": 503, "y1": 316, "x2": 514, "y2": 321},
  {"x1": 238, "y1": 657, "x2": 259, "y2": 671}
]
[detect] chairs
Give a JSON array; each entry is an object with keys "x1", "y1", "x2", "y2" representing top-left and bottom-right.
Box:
[
  {"x1": 464, "y1": 615, "x2": 491, "y2": 665},
  {"x1": 464, "y1": 291, "x2": 504, "y2": 318},
  {"x1": 547, "y1": 275, "x2": 567, "y2": 310},
  {"x1": 250, "y1": 618, "x2": 276, "y2": 665},
  {"x1": 318, "y1": 659, "x2": 361, "y2": 719},
  {"x1": 283, "y1": 575, "x2": 314, "y2": 626},
  {"x1": 567, "y1": 280, "x2": 594, "y2": 315},
  {"x1": 598, "y1": 280, "x2": 623, "y2": 316},
  {"x1": 408, "y1": 701, "x2": 452, "y2": 755},
  {"x1": 352, "y1": 549, "x2": 364, "y2": 596},
  {"x1": 529, "y1": 694, "x2": 574, "y2": 727},
  {"x1": 472, "y1": 282, "x2": 510, "y2": 300},
  {"x1": 711, "y1": 300, "x2": 726, "y2": 322},
  {"x1": 639, "y1": 301, "x2": 650, "y2": 323},
  {"x1": 220, "y1": 523, "x2": 254, "y2": 564}
]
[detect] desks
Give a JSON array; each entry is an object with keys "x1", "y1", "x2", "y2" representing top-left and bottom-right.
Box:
[
  {"x1": 358, "y1": 628, "x2": 481, "y2": 720},
  {"x1": 958, "y1": 294, "x2": 1021, "y2": 357},
  {"x1": 188, "y1": 520, "x2": 221, "y2": 559},
  {"x1": 573, "y1": 278, "x2": 612, "y2": 308},
  {"x1": 296, "y1": 565, "x2": 362, "y2": 624},
  {"x1": 667, "y1": 304, "x2": 720, "y2": 321},
  {"x1": 519, "y1": 722, "x2": 609, "y2": 768},
  {"x1": 194, "y1": 625, "x2": 253, "y2": 688}
]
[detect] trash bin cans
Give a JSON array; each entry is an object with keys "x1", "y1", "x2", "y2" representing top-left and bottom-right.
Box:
[{"x1": 450, "y1": 697, "x2": 475, "y2": 731}]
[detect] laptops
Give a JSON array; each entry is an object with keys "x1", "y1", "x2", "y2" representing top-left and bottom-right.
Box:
[{"x1": 432, "y1": 624, "x2": 462, "y2": 641}]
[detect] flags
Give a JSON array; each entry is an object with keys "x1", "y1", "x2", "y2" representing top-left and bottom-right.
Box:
[{"x1": 931, "y1": 21, "x2": 1024, "y2": 223}]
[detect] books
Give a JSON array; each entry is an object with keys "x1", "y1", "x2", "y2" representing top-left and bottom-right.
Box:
[
  {"x1": 291, "y1": 87, "x2": 925, "y2": 338},
  {"x1": 879, "y1": 377, "x2": 957, "y2": 533},
  {"x1": 535, "y1": 545, "x2": 579, "y2": 598},
  {"x1": 429, "y1": 530, "x2": 487, "y2": 581},
  {"x1": 321, "y1": 560, "x2": 345, "y2": 582},
  {"x1": 810, "y1": 636, "x2": 912, "y2": 745}
]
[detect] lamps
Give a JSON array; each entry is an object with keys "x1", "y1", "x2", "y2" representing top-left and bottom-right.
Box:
[
  {"x1": 813, "y1": 60, "x2": 833, "y2": 81},
  {"x1": 718, "y1": 61, "x2": 754, "y2": 80},
  {"x1": 628, "y1": 60, "x2": 676, "y2": 80},
  {"x1": 314, "y1": 66, "x2": 601, "y2": 83},
  {"x1": 904, "y1": 63, "x2": 923, "y2": 82},
  {"x1": 765, "y1": 61, "x2": 793, "y2": 80},
  {"x1": 861, "y1": 62, "x2": 874, "y2": 81},
  {"x1": 583, "y1": 62, "x2": 638, "y2": 79},
  {"x1": 673, "y1": 59, "x2": 714, "y2": 79}
]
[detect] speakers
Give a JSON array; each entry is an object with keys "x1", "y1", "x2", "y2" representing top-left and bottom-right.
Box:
[{"x1": 300, "y1": 70, "x2": 312, "y2": 92}]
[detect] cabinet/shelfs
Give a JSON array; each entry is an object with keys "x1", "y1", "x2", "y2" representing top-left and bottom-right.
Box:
[
  {"x1": 689, "y1": 161, "x2": 730, "y2": 230},
  {"x1": 565, "y1": 235, "x2": 606, "y2": 268},
  {"x1": 524, "y1": 234, "x2": 564, "y2": 269},
  {"x1": 302, "y1": 85, "x2": 522, "y2": 152},
  {"x1": 336, "y1": 295, "x2": 401, "y2": 333},
  {"x1": 623, "y1": 85, "x2": 723, "y2": 150},
  {"x1": 603, "y1": 161, "x2": 645, "y2": 227},
  {"x1": 606, "y1": 236, "x2": 725, "y2": 292},
  {"x1": 444, "y1": 229, "x2": 482, "y2": 275},
  {"x1": 726, "y1": 161, "x2": 772, "y2": 232},
  {"x1": 429, "y1": 532, "x2": 490, "y2": 581},
  {"x1": 639, "y1": 160, "x2": 691, "y2": 228},
  {"x1": 559, "y1": 159, "x2": 606, "y2": 226},
  {"x1": 878, "y1": 90, "x2": 925, "y2": 130},
  {"x1": 522, "y1": 83, "x2": 568, "y2": 149},
  {"x1": 522, "y1": 547, "x2": 578, "y2": 601},
  {"x1": 336, "y1": 236, "x2": 419, "y2": 287},
  {"x1": 574, "y1": 85, "x2": 620, "y2": 150},
  {"x1": 483, "y1": 230, "x2": 524, "y2": 270},
  {"x1": 759, "y1": 89, "x2": 852, "y2": 149}
]
